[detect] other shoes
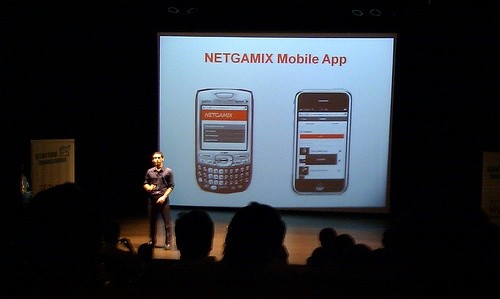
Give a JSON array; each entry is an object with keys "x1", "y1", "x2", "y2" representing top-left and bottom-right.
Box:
[
  {"x1": 164, "y1": 243, "x2": 171, "y2": 250},
  {"x1": 149, "y1": 241, "x2": 156, "y2": 247}
]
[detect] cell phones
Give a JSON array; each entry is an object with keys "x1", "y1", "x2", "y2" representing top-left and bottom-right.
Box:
[
  {"x1": 195, "y1": 88, "x2": 254, "y2": 193},
  {"x1": 292, "y1": 89, "x2": 352, "y2": 196}
]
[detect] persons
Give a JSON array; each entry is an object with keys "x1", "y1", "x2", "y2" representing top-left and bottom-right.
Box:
[
  {"x1": 27, "y1": 182, "x2": 308, "y2": 299},
  {"x1": 143, "y1": 151, "x2": 175, "y2": 250},
  {"x1": 307, "y1": 206, "x2": 500, "y2": 299}
]
